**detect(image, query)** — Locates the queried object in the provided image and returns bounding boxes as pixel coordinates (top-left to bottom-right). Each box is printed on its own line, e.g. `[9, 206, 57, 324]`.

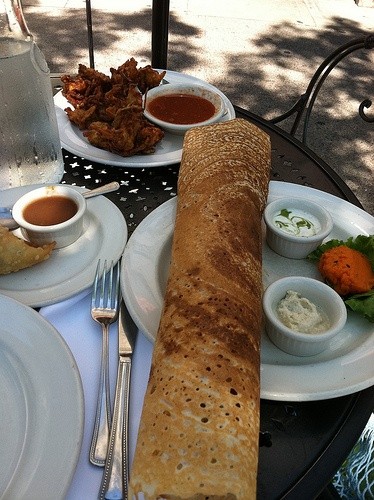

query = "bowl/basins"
[13, 186, 86, 248]
[142, 83, 224, 134]
[263, 277, 348, 356]
[263, 198, 334, 259]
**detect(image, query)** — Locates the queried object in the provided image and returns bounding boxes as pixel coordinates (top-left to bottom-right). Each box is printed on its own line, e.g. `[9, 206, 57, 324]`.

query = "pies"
[124, 118, 272, 500]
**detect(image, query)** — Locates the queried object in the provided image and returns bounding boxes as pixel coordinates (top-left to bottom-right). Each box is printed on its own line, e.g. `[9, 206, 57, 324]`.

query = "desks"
[48, 73, 374, 500]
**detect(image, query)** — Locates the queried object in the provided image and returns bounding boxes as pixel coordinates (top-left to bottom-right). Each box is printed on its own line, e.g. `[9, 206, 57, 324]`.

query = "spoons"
[0, 182, 120, 228]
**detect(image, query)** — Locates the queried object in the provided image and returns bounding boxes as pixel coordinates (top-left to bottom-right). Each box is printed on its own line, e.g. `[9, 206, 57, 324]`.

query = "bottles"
[0, 0, 64, 228]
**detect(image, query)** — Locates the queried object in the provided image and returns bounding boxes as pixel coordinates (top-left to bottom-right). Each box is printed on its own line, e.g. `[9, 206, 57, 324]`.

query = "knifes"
[97, 282, 138, 500]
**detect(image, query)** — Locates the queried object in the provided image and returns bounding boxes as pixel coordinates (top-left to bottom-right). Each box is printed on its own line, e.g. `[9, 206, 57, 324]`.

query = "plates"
[0, 294, 85, 500]
[0, 184, 127, 308]
[119, 180, 374, 400]
[52, 69, 236, 167]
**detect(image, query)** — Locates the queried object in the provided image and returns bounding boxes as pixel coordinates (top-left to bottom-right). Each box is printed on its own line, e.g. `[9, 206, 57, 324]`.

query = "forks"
[91, 259, 119, 466]
